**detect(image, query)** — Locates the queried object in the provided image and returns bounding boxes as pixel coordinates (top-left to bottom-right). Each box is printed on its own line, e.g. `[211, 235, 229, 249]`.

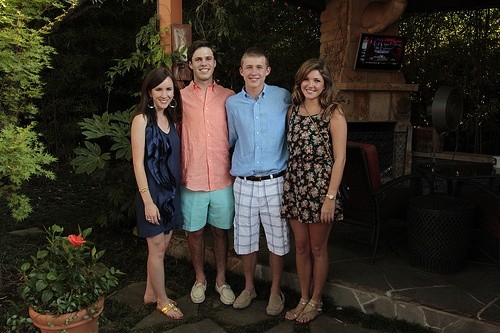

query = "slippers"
[143, 298, 177, 306]
[156, 303, 184, 320]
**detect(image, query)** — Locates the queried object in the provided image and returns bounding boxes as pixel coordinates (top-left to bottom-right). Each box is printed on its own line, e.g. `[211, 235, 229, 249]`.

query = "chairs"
[457, 180, 500, 267]
[333, 145, 415, 265]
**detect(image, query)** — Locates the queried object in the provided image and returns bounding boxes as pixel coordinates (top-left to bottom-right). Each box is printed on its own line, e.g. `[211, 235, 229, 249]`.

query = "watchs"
[138, 186, 151, 195]
[326, 192, 337, 201]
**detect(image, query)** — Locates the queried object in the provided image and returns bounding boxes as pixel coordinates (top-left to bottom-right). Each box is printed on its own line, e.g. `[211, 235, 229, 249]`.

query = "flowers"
[20, 223, 125, 324]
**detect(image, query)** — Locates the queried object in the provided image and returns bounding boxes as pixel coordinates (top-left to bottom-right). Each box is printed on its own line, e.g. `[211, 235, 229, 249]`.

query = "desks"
[427, 160, 495, 200]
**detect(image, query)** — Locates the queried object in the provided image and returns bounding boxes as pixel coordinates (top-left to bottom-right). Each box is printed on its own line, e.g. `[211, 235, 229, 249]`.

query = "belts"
[237, 169, 285, 181]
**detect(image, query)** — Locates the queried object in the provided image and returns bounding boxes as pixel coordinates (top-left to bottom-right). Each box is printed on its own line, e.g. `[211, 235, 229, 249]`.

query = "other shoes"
[215, 283, 235, 305]
[266, 292, 285, 316]
[233, 288, 256, 307]
[190, 280, 207, 303]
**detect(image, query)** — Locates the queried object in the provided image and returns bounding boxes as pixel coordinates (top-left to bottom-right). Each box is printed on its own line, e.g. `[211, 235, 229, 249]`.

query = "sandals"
[285, 298, 309, 320]
[296, 299, 323, 323]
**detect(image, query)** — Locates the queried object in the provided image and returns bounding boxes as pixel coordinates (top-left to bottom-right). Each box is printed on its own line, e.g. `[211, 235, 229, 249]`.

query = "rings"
[147, 219, 151, 221]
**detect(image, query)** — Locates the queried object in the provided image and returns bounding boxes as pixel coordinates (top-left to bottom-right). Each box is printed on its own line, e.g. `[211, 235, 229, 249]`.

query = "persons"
[179, 37, 237, 305]
[284, 57, 348, 324]
[130, 68, 184, 320]
[225, 49, 291, 315]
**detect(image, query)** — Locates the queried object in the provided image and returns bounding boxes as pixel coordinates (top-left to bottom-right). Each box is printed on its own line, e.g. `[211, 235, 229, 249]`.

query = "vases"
[29, 296, 104, 333]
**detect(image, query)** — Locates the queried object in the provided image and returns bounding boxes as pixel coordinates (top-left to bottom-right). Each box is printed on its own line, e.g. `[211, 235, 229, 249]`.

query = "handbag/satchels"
[340, 183, 351, 201]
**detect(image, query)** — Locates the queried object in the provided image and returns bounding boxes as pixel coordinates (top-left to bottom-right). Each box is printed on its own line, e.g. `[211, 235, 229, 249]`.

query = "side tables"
[407, 195, 478, 274]
